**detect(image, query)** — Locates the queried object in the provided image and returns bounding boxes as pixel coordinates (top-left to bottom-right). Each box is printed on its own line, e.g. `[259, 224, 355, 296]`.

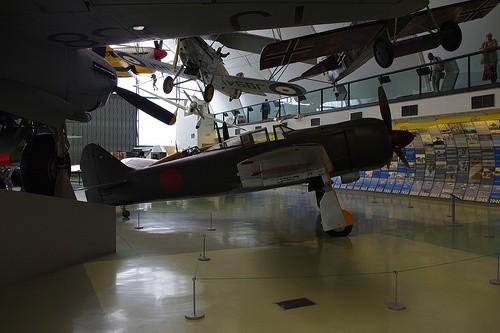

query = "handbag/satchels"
[440, 72, 445, 79]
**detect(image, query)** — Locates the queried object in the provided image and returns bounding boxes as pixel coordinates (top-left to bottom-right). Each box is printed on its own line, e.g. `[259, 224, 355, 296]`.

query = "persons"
[428, 53, 444, 93]
[260, 99, 271, 120]
[479, 33, 499, 84]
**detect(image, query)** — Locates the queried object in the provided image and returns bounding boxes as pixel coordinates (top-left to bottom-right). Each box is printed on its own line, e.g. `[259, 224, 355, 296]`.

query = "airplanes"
[86, 0, 500, 130]
[0, 0, 432, 285]
[80, 85, 418, 237]
